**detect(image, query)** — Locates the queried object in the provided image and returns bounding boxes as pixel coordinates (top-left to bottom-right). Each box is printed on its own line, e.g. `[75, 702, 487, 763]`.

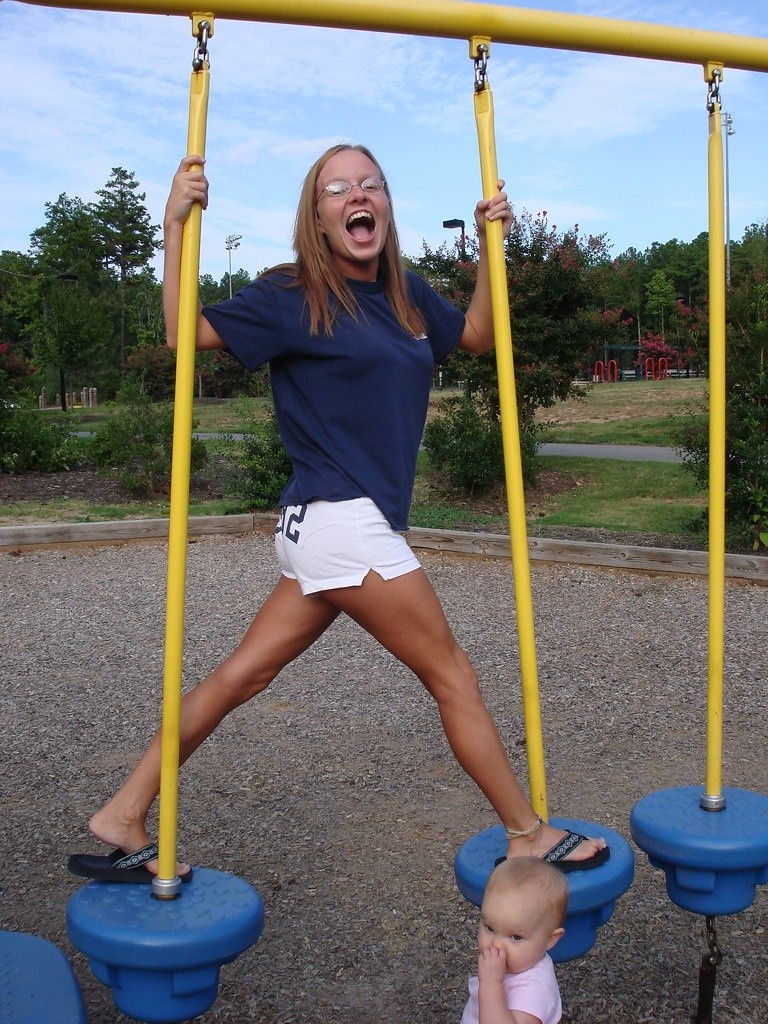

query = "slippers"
[493, 829, 611, 872]
[67, 843, 193, 883]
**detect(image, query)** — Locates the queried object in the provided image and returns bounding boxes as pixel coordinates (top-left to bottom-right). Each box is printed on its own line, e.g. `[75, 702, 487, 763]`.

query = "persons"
[457, 856, 570, 1024]
[67, 144, 611, 884]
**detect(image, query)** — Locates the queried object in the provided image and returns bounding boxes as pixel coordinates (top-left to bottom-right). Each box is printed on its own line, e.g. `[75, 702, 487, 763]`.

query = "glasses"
[314, 177, 394, 199]
